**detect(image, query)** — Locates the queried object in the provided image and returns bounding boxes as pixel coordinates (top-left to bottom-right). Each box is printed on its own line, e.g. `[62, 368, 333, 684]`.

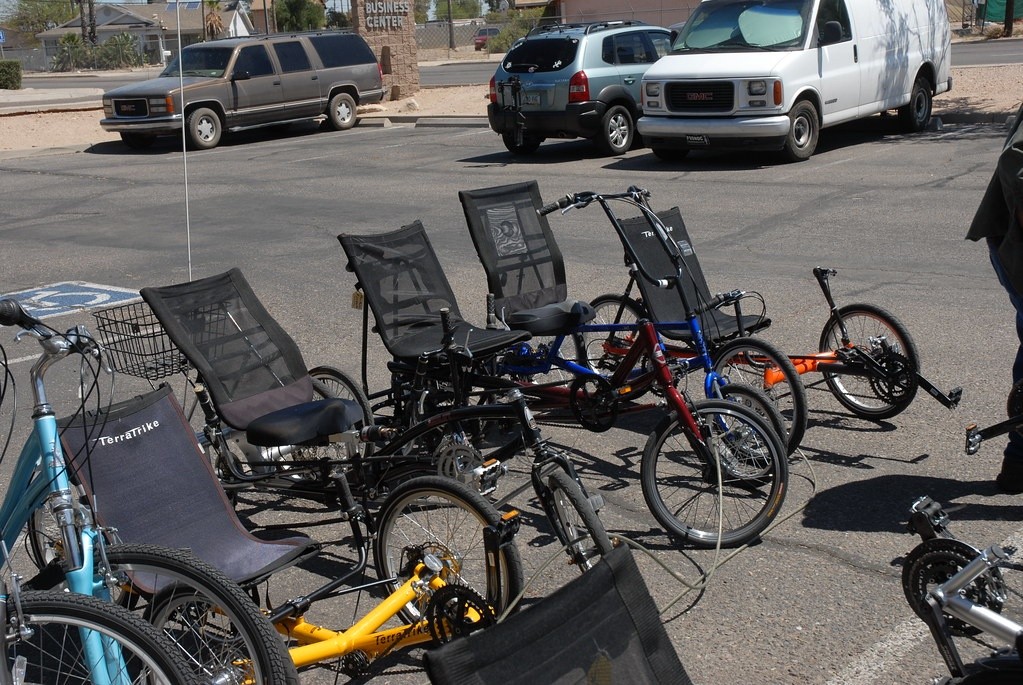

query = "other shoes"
[997, 457, 1023, 492]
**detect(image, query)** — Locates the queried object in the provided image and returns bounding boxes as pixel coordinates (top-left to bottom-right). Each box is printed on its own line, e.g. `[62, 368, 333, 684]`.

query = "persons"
[964, 103, 1023, 495]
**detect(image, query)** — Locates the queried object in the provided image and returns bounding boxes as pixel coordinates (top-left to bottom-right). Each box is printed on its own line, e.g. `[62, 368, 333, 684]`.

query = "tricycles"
[2, 185, 1023, 685]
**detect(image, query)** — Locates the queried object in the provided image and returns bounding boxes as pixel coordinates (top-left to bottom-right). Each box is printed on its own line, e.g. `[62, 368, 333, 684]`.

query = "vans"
[636, 0, 953, 164]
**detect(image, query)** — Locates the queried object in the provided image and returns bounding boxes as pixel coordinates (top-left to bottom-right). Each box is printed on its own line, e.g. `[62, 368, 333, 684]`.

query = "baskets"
[92, 301, 195, 381]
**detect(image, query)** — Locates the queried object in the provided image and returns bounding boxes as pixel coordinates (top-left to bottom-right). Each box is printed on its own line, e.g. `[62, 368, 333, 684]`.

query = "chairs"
[422, 540, 694, 685]
[56, 381, 322, 607]
[616, 206, 773, 347]
[336, 219, 533, 364]
[139, 265, 364, 448]
[616, 46, 635, 64]
[458, 180, 598, 337]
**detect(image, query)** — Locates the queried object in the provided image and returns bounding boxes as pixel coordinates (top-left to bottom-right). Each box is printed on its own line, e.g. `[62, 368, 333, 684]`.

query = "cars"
[650, 19, 704, 55]
[473, 27, 503, 51]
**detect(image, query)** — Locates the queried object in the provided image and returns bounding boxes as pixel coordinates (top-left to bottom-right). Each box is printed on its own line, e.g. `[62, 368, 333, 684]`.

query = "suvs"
[485, 16, 684, 159]
[99, 28, 386, 152]
